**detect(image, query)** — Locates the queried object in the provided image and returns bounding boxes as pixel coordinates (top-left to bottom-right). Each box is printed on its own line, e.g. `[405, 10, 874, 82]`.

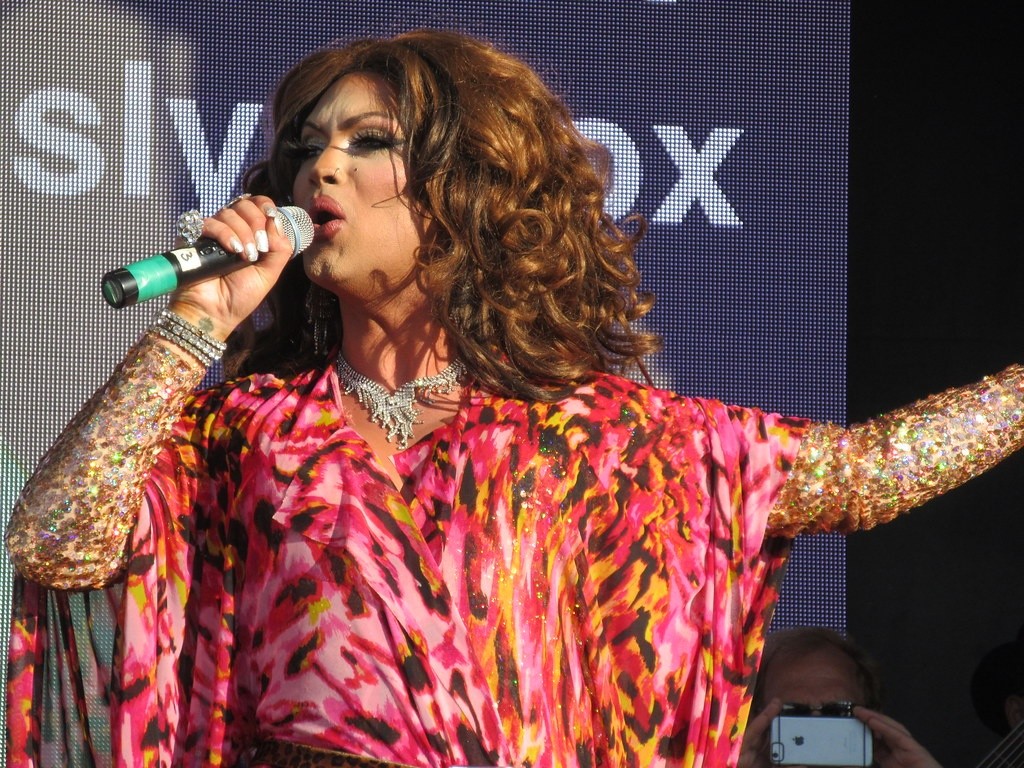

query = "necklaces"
[335, 349, 469, 450]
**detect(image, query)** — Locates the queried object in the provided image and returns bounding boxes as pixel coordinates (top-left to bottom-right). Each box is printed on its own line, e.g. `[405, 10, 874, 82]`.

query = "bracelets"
[149, 306, 229, 367]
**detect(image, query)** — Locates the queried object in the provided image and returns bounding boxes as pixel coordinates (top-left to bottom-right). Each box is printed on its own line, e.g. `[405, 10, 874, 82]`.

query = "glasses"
[779, 700, 855, 718]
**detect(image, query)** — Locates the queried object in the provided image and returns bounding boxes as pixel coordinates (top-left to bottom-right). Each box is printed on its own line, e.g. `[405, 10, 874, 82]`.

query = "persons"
[976, 638, 1024, 768]
[5, 26, 1024, 768]
[735, 627, 945, 768]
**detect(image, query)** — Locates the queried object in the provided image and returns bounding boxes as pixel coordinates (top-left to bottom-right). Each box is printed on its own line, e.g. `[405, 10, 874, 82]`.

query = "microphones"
[101, 206, 314, 308]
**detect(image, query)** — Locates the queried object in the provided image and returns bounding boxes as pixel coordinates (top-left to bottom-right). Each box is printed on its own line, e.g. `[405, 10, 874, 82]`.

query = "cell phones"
[769, 717, 875, 767]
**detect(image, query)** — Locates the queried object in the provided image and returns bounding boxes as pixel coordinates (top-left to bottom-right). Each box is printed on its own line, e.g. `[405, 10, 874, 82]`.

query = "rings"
[219, 191, 251, 208]
[176, 209, 205, 244]
